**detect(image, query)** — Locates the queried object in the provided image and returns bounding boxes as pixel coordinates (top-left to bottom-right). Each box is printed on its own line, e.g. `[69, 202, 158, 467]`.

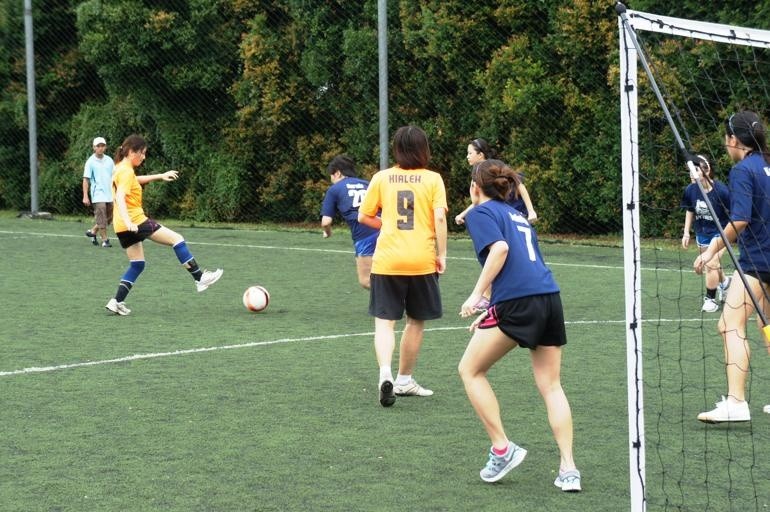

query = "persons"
[357, 125, 449, 407]
[321, 155, 382, 289]
[682, 153, 733, 313]
[458, 158, 581, 492]
[696, 111, 770, 425]
[105, 134, 223, 316]
[454, 137, 537, 310]
[82, 137, 115, 248]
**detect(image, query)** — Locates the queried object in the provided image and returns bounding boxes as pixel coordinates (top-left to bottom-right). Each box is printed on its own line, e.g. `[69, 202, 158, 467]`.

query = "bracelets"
[683, 235, 690, 239]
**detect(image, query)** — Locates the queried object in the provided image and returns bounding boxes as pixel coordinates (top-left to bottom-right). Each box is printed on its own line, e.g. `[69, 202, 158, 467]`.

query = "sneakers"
[105, 298, 132, 315]
[84, 229, 100, 247]
[554, 467, 583, 492]
[378, 374, 396, 407]
[717, 276, 734, 302]
[101, 239, 113, 247]
[194, 268, 224, 292]
[695, 397, 752, 425]
[701, 297, 719, 312]
[393, 377, 434, 397]
[480, 442, 528, 483]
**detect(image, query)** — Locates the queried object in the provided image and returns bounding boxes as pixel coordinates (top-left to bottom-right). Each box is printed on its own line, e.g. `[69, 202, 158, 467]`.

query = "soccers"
[243, 286, 271, 311]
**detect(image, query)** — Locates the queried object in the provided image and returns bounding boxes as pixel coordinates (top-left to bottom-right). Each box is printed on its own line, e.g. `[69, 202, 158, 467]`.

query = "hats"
[92, 136, 107, 146]
